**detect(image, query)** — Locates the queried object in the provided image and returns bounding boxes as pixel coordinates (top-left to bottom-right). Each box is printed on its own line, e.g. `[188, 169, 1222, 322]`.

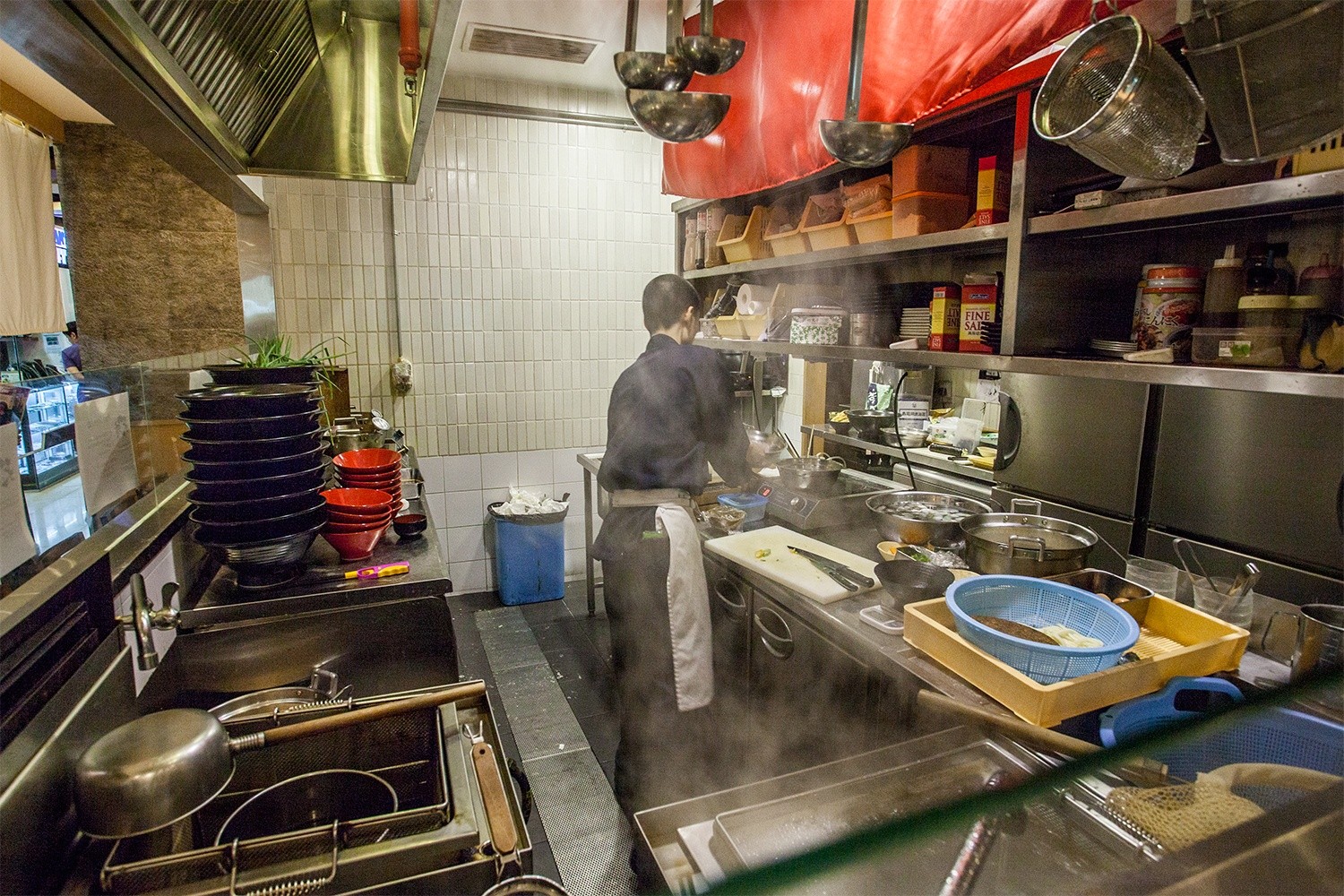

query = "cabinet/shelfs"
[670, 23, 1344, 398]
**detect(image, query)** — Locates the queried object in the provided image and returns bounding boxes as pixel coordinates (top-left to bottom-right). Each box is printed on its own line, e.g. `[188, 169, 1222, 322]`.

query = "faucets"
[935, 766, 1029, 896]
[127, 571, 182, 673]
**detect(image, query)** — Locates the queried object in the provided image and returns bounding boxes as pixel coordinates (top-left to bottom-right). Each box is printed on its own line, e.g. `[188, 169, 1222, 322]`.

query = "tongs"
[1173, 538, 1221, 607]
[1213, 562, 1262, 621]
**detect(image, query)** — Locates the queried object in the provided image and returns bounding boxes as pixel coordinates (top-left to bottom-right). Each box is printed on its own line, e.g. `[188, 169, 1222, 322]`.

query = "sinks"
[96, 701, 478, 896]
[144, 592, 461, 736]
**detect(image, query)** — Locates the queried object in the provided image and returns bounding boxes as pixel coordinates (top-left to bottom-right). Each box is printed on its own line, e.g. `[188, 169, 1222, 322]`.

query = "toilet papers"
[736, 283, 773, 316]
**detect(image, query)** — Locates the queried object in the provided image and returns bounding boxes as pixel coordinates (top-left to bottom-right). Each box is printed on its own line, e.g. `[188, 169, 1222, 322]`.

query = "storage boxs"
[930, 285, 960, 351]
[974, 155, 1012, 226]
[902, 566, 1251, 731]
[958, 282, 1000, 351]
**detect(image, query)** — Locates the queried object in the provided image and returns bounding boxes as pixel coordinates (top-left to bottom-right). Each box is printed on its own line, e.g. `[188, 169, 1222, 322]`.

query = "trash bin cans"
[487, 500, 570, 606]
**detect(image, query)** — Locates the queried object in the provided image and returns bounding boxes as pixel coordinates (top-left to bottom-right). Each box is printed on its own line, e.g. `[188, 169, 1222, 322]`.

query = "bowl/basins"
[1260, 605, 1344, 710]
[891, 145, 970, 239]
[829, 421, 852, 435]
[879, 428, 929, 448]
[1191, 327, 1285, 367]
[174, 362, 427, 588]
[874, 560, 956, 612]
[747, 430, 786, 464]
[844, 410, 897, 435]
[717, 492, 769, 523]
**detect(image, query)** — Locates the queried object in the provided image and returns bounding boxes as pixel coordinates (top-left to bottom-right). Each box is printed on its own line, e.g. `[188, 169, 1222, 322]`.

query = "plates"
[899, 308, 931, 346]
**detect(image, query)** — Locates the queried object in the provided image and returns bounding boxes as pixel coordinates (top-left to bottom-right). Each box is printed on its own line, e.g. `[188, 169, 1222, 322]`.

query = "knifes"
[787, 544, 875, 591]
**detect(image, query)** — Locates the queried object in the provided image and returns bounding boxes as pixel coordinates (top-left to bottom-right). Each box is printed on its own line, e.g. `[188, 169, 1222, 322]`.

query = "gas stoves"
[753, 472, 895, 537]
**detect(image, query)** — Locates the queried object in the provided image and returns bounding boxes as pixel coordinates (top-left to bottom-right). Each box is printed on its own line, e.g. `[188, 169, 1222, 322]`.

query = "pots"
[864, 492, 993, 545]
[73, 669, 486, 876]
[775, 458, 842, 492]
[323, 415, 386, 455]
[959, 498, 1097, 577]
[348, 405, 383, 425]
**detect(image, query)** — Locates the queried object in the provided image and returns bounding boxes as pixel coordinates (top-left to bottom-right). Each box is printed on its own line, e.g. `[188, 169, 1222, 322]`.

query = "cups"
[1124, 558, 1178, 601]
[1192, 576, 1254, 631]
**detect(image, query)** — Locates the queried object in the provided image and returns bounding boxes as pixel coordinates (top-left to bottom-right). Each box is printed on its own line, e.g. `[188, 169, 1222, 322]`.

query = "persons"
[61, 321, 84, 381]
[589, 273, 767, 827]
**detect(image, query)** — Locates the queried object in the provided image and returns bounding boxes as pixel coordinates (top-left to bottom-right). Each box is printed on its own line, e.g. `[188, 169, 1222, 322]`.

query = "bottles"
[1130, 263, 1200, 362]
[695, 206, 706, 269]
[704, 200, 727, 268]
[850, 313, 870, 346]
[683, 209, 697, 271]
[1200, 242, 1341, 367]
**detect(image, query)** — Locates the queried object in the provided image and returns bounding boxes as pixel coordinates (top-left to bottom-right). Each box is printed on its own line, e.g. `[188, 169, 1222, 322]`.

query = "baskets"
[1100, 676, 1344, 818]
[944, 573, 1141, 686]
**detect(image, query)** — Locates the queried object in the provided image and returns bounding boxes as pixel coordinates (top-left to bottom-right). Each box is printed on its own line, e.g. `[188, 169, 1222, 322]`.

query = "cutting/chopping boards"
[705, 525, 884, 604]
